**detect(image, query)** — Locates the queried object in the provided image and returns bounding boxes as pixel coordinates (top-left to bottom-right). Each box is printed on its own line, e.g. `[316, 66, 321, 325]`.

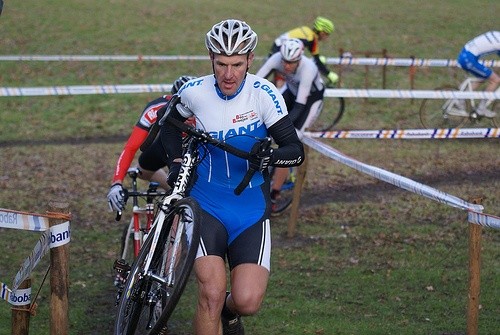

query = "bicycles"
[114, 80, 346, 302]
[112, 95, 274, 334]
[419, 62, 500, 129]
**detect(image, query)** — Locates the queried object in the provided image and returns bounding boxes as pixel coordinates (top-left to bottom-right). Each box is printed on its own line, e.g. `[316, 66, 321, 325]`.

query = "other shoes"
[271, 198, 279, 213]
[154, 306, 168, 335]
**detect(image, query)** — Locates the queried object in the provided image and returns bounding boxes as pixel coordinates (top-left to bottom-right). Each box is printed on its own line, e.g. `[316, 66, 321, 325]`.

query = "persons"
[252, 37, 325, 204]
[267, 16, 339, 84]
[457, 30, 500, 118]
[156, 18, 305, 335]
[106, 76, 200, 214]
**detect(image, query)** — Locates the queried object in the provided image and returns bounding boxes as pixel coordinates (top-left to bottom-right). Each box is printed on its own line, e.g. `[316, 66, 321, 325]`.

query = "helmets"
[205, 20, 258, 54]
[280, 37, 304, 61]
[313, 16, 334, 34]
[171, 75, 198, 95]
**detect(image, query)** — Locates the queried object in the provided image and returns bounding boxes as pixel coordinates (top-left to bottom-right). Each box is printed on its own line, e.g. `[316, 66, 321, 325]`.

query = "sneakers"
[475, 107, 497, 118]
[217, 291, 245, 335]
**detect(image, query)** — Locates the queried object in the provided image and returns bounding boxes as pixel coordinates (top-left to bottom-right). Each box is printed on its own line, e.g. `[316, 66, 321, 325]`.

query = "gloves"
[247, 142, 274, 171]
[328, 70, 339, 84]
[319, 55, 326, 64]
[106, 183, 127, 215]
[167, 161, 199, 192]
